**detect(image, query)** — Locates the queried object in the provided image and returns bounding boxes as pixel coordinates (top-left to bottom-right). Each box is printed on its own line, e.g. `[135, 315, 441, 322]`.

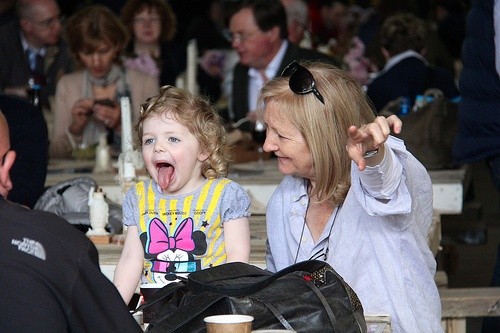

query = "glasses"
[280, 58, 325, 105]
[23, 15, 64, 28]
[222, 29, 270, 43]
[133, 17, 162, 24]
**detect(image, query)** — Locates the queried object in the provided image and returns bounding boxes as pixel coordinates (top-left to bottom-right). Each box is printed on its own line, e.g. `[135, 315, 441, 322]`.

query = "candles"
[186, 40, 197, 94]
[120, 95, 135, 177]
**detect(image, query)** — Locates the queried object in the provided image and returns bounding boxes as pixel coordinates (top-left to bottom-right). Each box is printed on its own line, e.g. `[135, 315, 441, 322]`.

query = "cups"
[204, 315, 254, 333]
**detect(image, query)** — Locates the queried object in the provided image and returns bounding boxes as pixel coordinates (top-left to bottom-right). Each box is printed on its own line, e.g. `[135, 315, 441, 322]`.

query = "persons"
[0, 0, 499, 333]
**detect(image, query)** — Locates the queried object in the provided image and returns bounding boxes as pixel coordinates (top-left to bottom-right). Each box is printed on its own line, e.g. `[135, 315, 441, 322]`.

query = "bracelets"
[363, 146, 380, 159]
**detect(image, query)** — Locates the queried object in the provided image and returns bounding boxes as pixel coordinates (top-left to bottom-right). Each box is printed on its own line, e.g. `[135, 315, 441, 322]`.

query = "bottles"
[398, 94, 433, 116]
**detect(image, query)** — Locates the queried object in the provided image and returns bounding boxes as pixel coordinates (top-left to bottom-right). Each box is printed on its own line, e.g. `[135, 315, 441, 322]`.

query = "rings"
[104, 117, 110, 125]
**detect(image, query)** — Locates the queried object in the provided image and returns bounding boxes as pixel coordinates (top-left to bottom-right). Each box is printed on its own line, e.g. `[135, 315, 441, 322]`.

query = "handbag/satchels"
[141, 260, 367, 333]
[376, 87, 463, 171]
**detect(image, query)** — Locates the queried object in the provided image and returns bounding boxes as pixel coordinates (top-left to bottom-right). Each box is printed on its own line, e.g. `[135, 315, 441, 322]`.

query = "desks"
[35, 161, 464, 216]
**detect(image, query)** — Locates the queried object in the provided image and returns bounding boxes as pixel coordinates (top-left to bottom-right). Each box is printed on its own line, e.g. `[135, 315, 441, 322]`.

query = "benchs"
[439, 288, 500, 333]
